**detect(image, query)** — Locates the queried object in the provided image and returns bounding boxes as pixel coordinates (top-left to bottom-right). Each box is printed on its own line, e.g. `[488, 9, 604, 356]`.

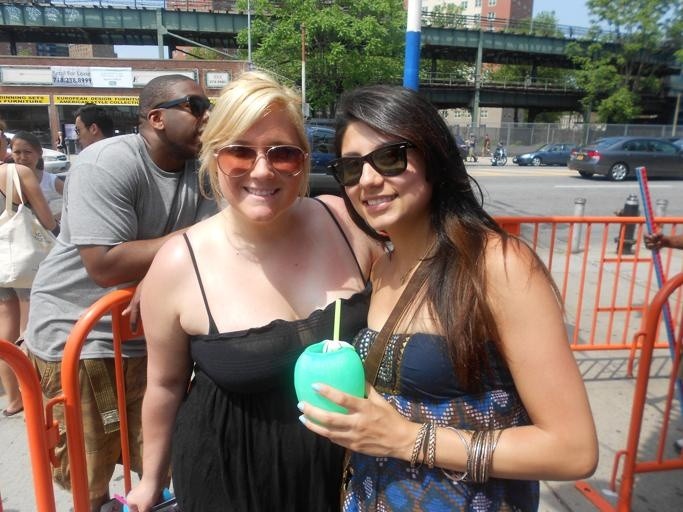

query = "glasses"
[153, 93, 214, 118]
[209, 141, 309, 185]
[73, 126, 84, 134]
[325, 140, 414, 187]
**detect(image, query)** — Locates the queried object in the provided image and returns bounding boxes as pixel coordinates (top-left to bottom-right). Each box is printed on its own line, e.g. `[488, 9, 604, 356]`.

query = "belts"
[76, 354, 129, 437]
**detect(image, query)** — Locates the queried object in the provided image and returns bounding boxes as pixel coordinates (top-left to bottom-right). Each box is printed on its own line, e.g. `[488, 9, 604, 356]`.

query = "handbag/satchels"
[0, 160, 58, 292]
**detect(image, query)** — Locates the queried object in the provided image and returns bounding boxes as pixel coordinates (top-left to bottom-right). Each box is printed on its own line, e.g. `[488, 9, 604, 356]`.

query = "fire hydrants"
[612, 191, 646, 257]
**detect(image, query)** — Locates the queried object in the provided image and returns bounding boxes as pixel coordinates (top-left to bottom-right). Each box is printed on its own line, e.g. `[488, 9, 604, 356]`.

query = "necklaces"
[390, 228, 441, 286]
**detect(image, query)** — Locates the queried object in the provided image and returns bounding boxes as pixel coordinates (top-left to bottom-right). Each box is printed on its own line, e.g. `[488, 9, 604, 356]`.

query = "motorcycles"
[490, 143, 509, 168]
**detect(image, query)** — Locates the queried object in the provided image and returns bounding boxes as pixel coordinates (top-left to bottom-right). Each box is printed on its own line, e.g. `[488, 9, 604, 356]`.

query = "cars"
[303, 124, 344, 198]
[512, 141, 584, 169]
[566, 135, 683, 184]
[657, 135, 683, 150]
[4, 131, 73, 175]
[454, 134, 467, 159]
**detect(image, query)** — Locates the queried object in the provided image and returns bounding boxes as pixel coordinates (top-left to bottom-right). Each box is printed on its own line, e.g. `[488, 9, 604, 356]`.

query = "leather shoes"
[1, 405, 27, 420]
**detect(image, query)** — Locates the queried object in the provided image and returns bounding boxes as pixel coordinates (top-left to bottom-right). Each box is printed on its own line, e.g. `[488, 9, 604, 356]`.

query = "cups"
[293, 342, 367, 427]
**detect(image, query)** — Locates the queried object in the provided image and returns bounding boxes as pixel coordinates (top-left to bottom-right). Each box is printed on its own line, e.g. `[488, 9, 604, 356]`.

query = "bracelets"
[410, 422, 509, 486]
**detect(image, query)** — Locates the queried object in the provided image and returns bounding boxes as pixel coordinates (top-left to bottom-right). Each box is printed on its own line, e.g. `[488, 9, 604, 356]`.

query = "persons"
[481, 133, 493, 157]
[0, 96, 120, 420]
[126, 64, 391, 512]
[24, 74, 222, 512]
[466, 133, 478, 164]
[643, 231, 683, 451]
[300, 84, 599, 512]
